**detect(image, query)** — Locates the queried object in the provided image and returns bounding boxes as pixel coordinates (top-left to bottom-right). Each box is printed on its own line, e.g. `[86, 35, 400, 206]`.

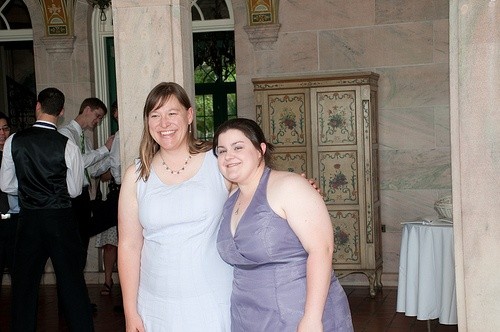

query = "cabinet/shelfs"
[250, 71, 384, 299]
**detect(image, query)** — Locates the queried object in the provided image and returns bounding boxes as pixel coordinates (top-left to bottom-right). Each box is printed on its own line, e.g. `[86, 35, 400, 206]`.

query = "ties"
[81, 130, 91, 186]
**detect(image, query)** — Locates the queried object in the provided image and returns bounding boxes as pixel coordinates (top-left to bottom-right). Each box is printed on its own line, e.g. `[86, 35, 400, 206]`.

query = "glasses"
[0, 126, 10, 131]
[94, 110, 103, 119]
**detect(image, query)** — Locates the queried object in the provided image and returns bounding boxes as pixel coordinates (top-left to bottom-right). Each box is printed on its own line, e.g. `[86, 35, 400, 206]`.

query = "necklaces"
[235, 188, 252, 215]
[159, 151, 191, 174]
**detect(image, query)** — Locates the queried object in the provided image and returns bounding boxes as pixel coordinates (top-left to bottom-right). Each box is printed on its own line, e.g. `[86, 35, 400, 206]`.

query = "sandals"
[100, 278, 113, 297]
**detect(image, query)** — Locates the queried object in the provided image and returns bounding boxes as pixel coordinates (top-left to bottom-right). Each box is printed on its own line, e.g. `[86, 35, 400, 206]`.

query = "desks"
[396, 215, 459, 332]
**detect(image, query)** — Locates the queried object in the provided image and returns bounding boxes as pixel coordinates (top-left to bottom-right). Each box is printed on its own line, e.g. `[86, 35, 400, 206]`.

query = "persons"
[212, 117, 354, 332]
[53, 96, 115, 318]
[118, 83, 324, 332]
[2, 88, 95, 332]
[95, 166, 117, 295]
[0, 114, 18, 304]
[91, 99, 121, 185]
